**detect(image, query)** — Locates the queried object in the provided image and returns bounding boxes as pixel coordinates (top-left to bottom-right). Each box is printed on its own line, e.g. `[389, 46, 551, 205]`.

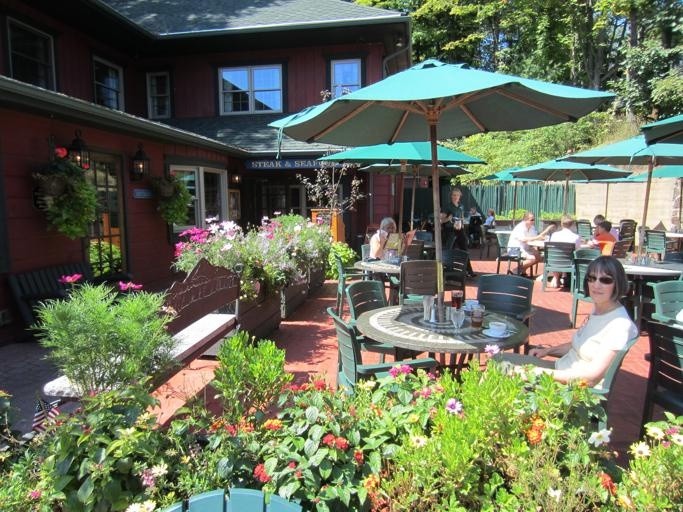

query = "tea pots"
[450, 216, 461, 230]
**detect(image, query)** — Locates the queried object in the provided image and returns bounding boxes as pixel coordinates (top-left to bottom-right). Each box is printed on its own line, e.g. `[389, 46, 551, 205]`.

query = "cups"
[489, 321, 506, 336]
[445, 306, 456, 321]
[421, 295, 434, 323]
[450, 290, 463, 308]
[465, 300, 478, 310]
[471, 304, 485, 328]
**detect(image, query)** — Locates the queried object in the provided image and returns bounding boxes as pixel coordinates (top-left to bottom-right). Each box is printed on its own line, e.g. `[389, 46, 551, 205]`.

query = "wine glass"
[450, 309, 465, 340]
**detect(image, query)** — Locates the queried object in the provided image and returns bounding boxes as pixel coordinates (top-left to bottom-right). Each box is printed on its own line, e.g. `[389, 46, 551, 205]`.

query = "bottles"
[427, 303, 437, 323]
[388, 250, 396, 264]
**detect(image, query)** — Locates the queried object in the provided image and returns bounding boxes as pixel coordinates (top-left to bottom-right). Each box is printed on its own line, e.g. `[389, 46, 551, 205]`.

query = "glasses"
[530, 219, 535, 221]
[587, 274, 616, 284]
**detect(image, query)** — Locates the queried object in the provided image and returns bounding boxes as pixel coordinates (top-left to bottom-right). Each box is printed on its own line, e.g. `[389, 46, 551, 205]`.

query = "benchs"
[5, 260, 128, 342]
[39, 256, 241, 400]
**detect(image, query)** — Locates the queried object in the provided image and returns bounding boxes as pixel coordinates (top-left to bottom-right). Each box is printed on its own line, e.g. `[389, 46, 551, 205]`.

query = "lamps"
[69, 129, 91, 169]
[128, 142, 151, 181]
[226, 167, 243, 189]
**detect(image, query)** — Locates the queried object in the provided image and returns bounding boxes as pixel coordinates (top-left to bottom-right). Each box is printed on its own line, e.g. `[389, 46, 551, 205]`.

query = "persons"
[507, 213, 619, 289]
[478, 254, 639, 418]
[439, 187, 477, 279]
[368, 216, 404, 307]
[405, 205, 496, 260]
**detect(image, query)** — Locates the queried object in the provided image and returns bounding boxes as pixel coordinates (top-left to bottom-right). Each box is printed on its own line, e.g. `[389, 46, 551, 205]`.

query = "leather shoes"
[466, 273, 477, 278]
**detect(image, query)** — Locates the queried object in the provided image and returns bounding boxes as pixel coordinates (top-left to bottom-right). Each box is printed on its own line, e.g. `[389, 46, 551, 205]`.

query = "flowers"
[155, 169, 195, 226]
[166, 204, 337, 295]
[32, 140, 98, 242]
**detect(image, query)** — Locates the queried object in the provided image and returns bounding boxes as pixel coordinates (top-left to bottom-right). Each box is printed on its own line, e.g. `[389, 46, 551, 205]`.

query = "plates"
[461, 306, 472, 312]
[482, 328, 511, 339]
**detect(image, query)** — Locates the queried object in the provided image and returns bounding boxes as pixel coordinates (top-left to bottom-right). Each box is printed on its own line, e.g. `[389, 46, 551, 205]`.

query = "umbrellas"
[315, 138, 490, 256]
[357, 162, 473, 231]
[265, 56, 621, 362]
